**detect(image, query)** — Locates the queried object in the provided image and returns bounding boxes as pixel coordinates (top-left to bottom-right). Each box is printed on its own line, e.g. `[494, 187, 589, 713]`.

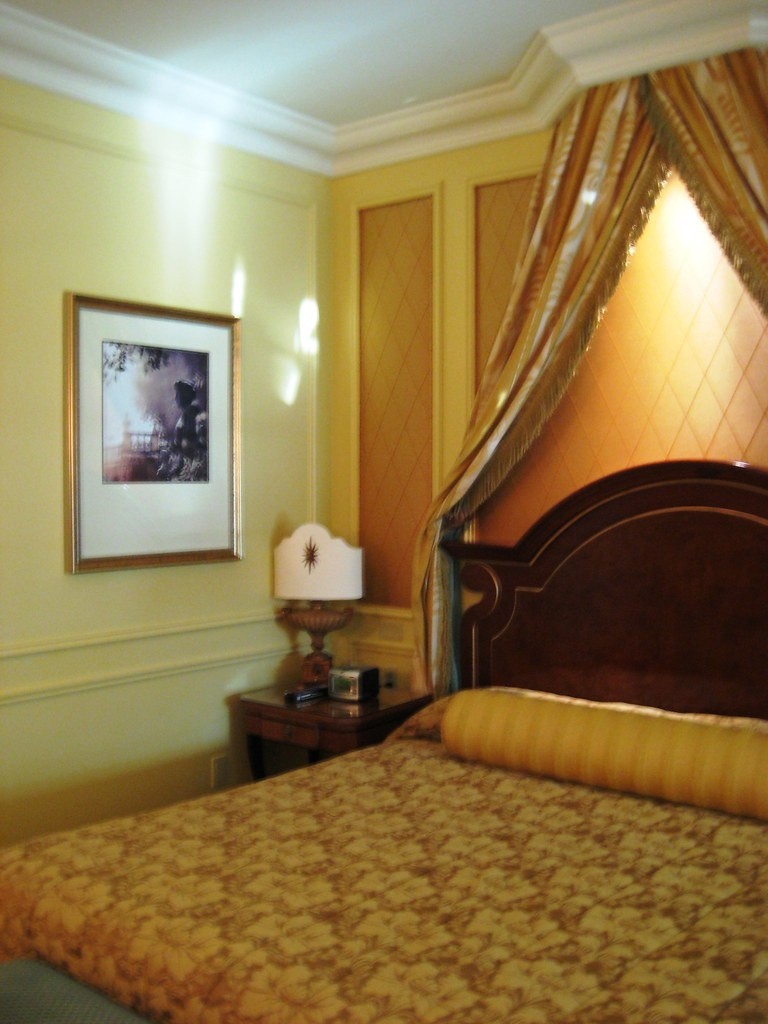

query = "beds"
[0, 456, 767, 1024]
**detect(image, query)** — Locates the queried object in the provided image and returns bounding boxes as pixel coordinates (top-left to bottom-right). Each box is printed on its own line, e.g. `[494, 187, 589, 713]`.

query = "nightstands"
[240, 682, 433, 781]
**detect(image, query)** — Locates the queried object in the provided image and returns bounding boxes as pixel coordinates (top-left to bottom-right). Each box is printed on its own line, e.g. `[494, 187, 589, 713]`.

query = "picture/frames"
[62, 291, 243, 577]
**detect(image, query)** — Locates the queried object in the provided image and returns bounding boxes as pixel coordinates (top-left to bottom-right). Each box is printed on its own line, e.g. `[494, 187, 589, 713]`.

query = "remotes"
[284, 685, 328, 704]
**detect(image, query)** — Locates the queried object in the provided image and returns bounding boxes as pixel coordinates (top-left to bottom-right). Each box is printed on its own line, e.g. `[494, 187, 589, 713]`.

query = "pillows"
[441, 685, 768, 823]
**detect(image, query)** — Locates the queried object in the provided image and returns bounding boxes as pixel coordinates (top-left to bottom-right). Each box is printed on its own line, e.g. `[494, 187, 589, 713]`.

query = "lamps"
[274, 523, 364, 683]
